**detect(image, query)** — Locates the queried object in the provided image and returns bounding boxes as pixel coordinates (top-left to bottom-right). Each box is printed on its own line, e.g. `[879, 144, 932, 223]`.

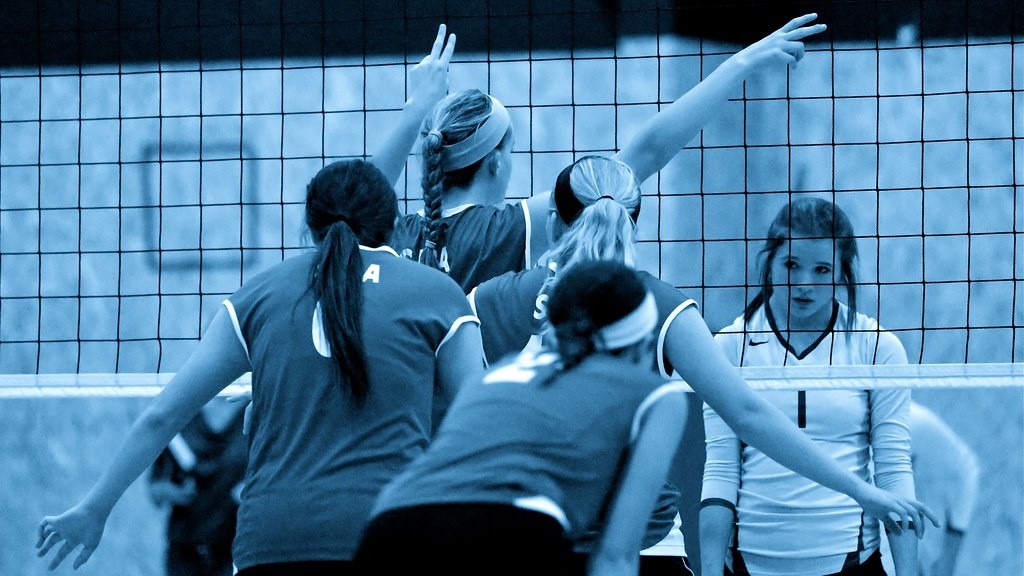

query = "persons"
[35, 159, 483, 576]
[698, 199, 919, 576]
[910, 400, 982, 575]
[350, 260, 687, 576]
[368, 12, 827, 295]
[467, 154, 941, 576]
[147, 398, 250, 576]
